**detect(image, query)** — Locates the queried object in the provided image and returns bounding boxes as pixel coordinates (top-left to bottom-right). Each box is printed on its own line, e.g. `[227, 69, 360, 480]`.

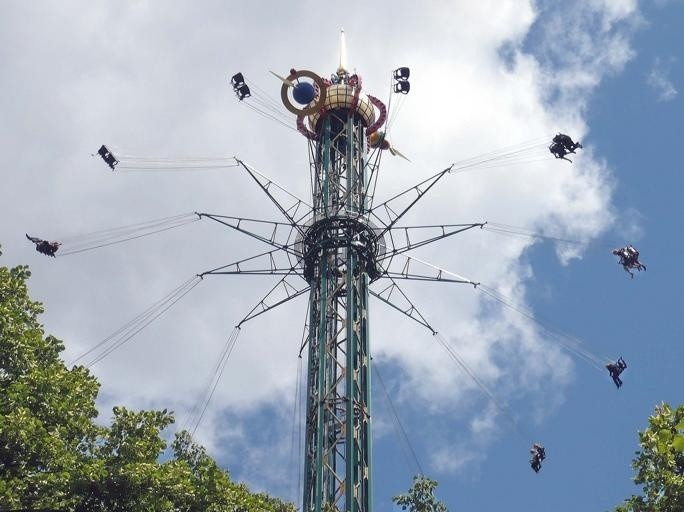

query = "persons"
[613, 244, 646, 278]
[549, 144, 572, 162]
[553, 134, 583, 152]
[605, 363, 623, 389]
[529, 443, 545, 473]
[25, 234, 59, 258]
